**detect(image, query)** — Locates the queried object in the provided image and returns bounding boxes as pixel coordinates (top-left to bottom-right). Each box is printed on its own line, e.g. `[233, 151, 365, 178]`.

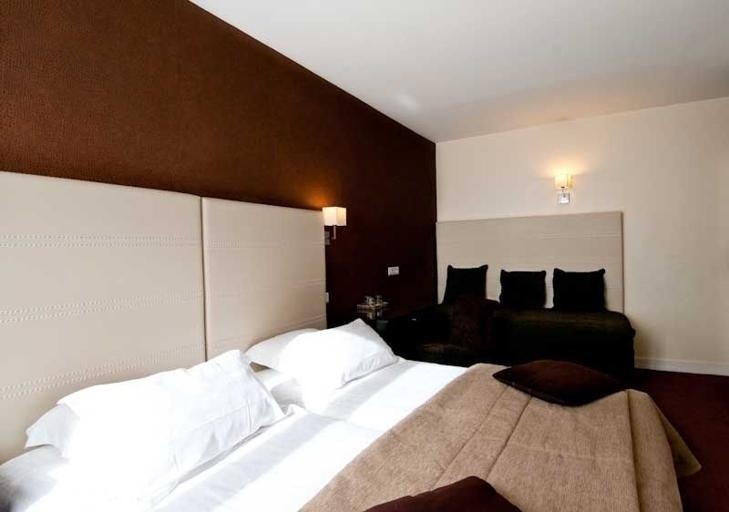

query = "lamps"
[322, 206, 347, 245]
[554, 174, 572, 204]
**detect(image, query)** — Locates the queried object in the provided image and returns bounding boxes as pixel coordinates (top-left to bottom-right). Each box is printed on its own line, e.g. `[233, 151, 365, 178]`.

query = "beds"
[0, 361, 701, 512]
[0, 170, 702, 512]
[383, 212, 636, 385]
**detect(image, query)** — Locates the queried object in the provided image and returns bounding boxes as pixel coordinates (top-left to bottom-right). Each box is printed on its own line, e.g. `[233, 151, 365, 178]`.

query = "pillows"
[442, 265, 488, 303]
[279, 318, 400, 409]
[243, 328, 320, 372]
[24, 368, 186, 483]
[57, 350, 293, 494]
[499, 270, 546, 308]
[552, 269, 609, 311]
